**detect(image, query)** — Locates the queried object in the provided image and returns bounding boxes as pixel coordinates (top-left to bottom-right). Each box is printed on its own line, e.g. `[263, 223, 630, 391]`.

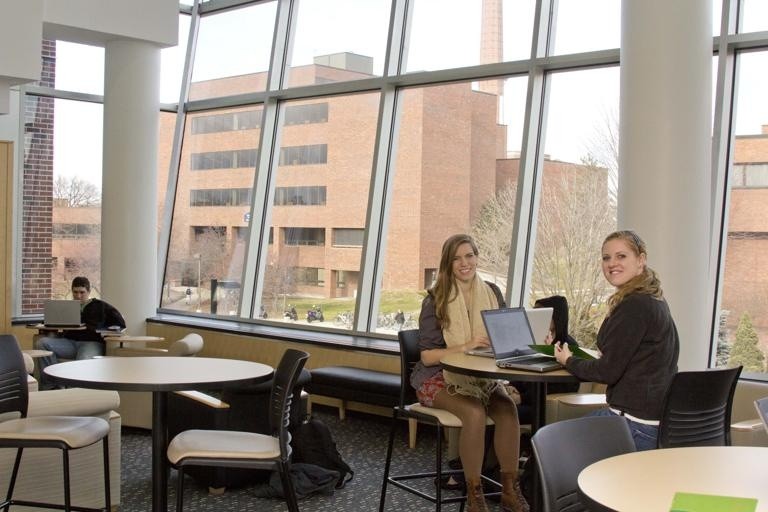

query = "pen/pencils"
[555, 344, 561, 352]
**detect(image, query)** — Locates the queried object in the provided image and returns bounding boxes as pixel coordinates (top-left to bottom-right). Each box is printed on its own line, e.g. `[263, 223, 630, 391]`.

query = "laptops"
[464, 307, 554, 358]
[480, 307, 563, 372]
[44, 299, 86, 327]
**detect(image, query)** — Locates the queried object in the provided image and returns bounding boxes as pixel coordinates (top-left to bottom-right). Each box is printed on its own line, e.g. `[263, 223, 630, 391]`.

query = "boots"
[500, 471, 530, 512]
[466, 481, 489, 512]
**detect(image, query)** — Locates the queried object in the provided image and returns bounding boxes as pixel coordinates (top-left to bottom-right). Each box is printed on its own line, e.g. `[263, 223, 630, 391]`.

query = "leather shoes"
[435, 457, 461, 489]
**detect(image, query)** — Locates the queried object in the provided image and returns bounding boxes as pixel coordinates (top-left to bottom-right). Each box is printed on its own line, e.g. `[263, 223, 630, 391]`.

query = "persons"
[519, 230, 684, 512]
[433, 296, 582, 495]
[394, 308, 405, 331]
[405, 234, 533, 512]
[32, 276, 129, 391]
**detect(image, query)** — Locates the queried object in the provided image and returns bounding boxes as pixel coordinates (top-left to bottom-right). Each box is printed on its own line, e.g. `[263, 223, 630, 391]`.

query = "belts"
[609, 407, 661, 425]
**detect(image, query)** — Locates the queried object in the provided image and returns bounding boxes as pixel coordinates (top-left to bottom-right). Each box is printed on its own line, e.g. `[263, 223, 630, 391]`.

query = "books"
[95, 325, 127, 338]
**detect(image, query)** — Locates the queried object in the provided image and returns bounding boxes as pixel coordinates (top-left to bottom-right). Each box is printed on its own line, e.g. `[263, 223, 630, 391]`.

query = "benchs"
[304, 364, 418, 454]
